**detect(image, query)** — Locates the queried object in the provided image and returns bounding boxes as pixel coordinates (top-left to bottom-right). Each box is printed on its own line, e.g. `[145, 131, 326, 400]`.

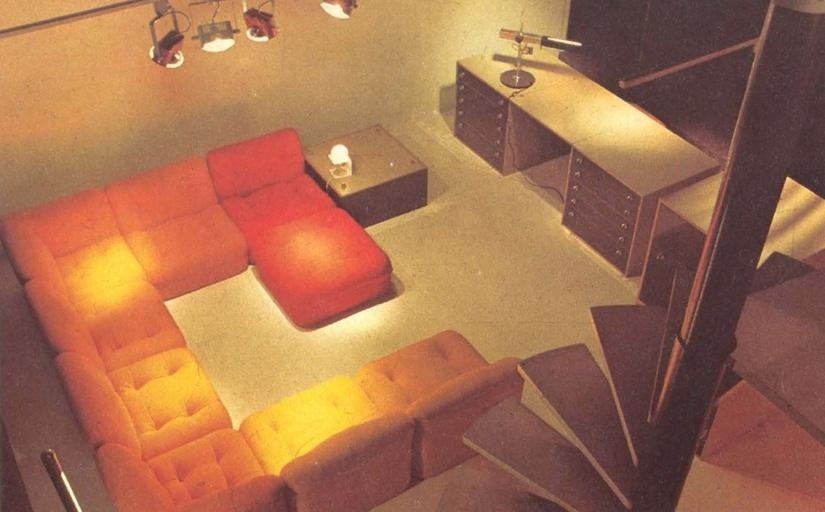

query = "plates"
[305, 126, 429, 229]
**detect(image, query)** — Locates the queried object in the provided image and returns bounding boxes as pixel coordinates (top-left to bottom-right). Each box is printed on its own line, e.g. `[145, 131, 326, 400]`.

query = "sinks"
[0, 129, 524, 512]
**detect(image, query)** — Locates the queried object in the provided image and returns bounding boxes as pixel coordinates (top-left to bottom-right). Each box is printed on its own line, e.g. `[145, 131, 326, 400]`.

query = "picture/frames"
[440, 52, 717, 280]
[635, 174, 825, 313]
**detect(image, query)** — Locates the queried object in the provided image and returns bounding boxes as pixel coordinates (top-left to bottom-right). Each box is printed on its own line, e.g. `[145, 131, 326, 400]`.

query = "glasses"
[320, 2, 355, 24]
[242, 1, 275, 46]
[142, 1, 186, 74]
[327, 144, 353, 181]
[189, 0, 242, 58]
